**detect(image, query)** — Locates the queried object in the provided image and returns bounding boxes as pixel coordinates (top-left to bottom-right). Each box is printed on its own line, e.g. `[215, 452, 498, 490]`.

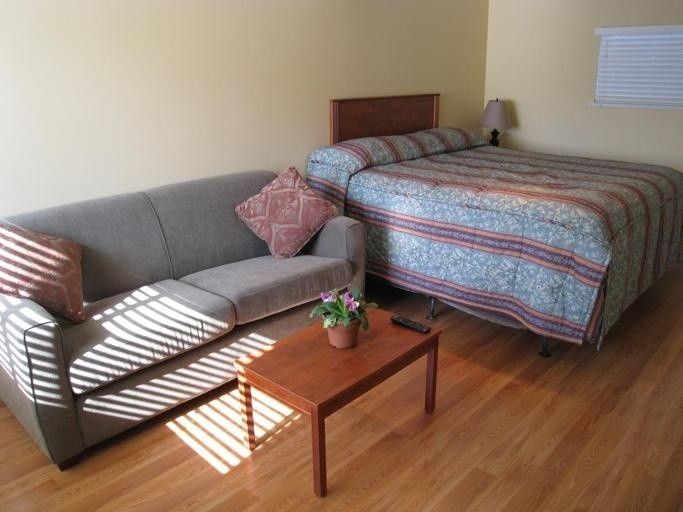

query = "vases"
[328, 319, 361, 349]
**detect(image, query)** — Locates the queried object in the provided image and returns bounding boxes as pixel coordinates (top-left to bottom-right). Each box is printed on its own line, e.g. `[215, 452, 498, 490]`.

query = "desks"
[233, 307, 442, 497]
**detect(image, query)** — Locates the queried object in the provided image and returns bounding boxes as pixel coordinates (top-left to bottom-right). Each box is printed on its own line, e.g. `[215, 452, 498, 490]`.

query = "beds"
[307, 92, 683, 358]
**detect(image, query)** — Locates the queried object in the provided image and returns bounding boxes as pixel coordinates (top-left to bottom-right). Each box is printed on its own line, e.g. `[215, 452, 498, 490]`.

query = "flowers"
[310, 288, 378, 330]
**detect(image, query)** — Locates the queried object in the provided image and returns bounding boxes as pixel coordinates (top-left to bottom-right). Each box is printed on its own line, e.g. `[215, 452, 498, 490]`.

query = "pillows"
[234, 166, 339, 258]
[1, 219, 86, 323]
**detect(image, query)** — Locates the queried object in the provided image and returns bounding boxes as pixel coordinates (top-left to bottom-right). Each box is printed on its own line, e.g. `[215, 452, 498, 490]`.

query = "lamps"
[477, 98, 512, 146]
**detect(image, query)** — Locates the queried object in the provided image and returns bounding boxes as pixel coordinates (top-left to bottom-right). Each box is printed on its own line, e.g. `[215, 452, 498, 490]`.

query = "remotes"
[390, 313, 431, 334]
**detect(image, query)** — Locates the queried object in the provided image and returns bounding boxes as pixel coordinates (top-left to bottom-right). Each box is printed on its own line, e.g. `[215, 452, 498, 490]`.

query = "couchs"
[0, 170, 367, 472]
